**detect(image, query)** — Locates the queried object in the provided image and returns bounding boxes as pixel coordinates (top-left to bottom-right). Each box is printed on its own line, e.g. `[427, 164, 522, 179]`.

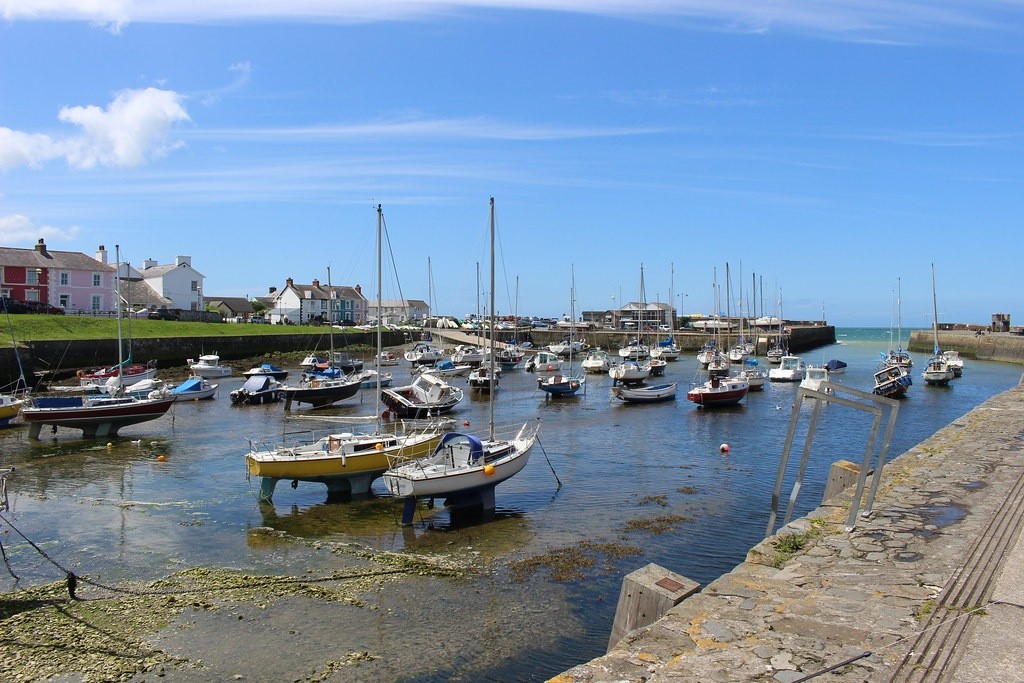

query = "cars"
[603, 323, 638, 332]
[147, 309, 183, 321]
[462, 312, 561, 332]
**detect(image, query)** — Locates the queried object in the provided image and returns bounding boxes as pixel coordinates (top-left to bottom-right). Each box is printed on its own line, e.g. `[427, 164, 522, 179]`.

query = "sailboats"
[0, 284, 32, 431]
[525, 263, 590, 356]
[688, 261, 794, 408]
[608, 263, 653, 382]
[822, 302, 847, 373]
[246, 205, 452, 479]
[581, 307, 618, 374]
[21, 243, 184, 438]
[921, 262, 956, 386]
[619, 262, 682, 378]
[872, 277, 915, 400]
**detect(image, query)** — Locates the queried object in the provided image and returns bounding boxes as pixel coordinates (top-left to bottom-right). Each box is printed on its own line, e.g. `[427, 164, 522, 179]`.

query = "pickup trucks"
[224, 315, 270, 324]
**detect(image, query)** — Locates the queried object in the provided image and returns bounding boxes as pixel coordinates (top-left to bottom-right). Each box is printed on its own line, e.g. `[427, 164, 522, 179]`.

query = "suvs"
[659, 325, 671, 333]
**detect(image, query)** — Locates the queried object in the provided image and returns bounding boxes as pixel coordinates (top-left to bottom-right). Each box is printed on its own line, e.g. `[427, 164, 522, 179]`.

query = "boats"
[374, 195, 537, 499]
[381, 373, 465, 421]
[147, 375, 215, 404]
[242, 364, 289, 381]
[942, 350, 963, 377]
[524, 350, 586, 405]
[612, 381, 677, 403]
[187, 345, 233, 379]
[230, 375, 285, 406]
[769, 353, 808, 382]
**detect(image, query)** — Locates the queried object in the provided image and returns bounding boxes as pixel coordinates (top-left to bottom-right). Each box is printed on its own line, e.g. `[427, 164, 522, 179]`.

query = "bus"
[620, 320, 661, 330]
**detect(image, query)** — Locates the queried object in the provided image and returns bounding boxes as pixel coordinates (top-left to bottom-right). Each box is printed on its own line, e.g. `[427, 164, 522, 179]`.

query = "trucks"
[556, 316, 591, 331]
